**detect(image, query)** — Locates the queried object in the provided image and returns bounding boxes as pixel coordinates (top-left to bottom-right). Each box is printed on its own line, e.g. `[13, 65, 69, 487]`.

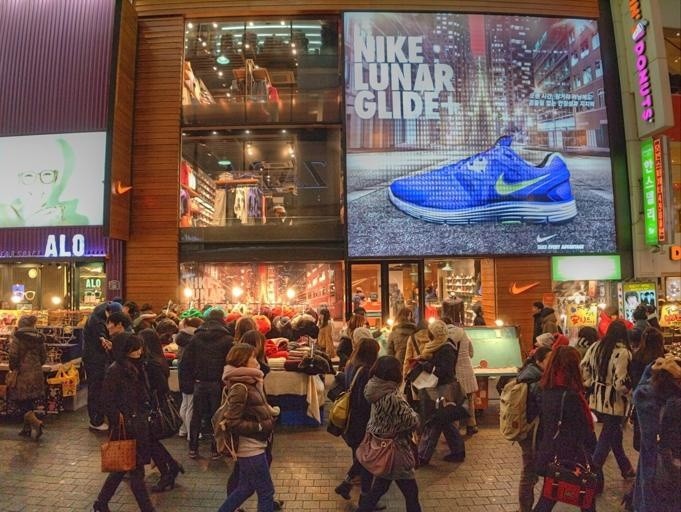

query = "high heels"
[168, 462, 185, 477]
[152, 475, 174, 491]
[93, 501, 110, 512]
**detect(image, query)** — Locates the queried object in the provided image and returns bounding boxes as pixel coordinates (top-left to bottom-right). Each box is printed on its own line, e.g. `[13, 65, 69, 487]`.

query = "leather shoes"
[89, 423, 109, 431]
[443, 452, 465, 461]
[467, 426, 478, 435]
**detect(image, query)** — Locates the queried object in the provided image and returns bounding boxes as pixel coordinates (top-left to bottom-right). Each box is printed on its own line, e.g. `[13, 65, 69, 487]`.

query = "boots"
[335, 481, 352, 499]
[26, 412, 44, 439]
[19, 416, 31, 436]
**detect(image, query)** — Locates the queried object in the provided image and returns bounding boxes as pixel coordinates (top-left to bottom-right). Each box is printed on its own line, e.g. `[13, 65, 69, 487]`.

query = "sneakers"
[388, 135, 578, 226]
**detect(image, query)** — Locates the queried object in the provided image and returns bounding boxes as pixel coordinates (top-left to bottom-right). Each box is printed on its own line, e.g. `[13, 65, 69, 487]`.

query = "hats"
[18, 315, 36, 329]
[428, 320, 448, 339]
[536, 333, 554, 346]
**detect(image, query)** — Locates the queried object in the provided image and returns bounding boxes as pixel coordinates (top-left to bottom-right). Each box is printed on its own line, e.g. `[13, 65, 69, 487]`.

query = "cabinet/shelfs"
[0, 324, 88, 412]
[445, 276, 477, 327]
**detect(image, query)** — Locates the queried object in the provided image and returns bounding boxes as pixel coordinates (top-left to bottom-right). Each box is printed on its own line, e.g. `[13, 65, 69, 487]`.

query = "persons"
[5, 314, 47, 442]
[621, 357, 681, 512]
[327, 337, 386, 510]
[93, 334, 155, 512]
[83, 297, 334, 459]
[211, 343, 281, 512]
[356, 356, 422, 512]
[531, 297, 665, 493]
[227, 330, 284, 512]
[534, 346, 598, 512]
[334, 283, 486, 468]
[500, 347, 555, 512]
[137, 328, 185, 492]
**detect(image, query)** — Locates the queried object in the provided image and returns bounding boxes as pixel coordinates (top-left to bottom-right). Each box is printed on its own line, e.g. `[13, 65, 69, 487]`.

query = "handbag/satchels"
[412, 366, 471, 425]
[5, 368, 19, 389]
[101, 413, 137, 472]
[330, 391, 351, 428]
[543, 459, 598, 509]
[355, 431, 395, 476]
[150, 389, 183, 440]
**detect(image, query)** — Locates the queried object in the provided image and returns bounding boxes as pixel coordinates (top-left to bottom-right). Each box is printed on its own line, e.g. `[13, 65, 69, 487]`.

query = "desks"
[169, 369, 327, 426]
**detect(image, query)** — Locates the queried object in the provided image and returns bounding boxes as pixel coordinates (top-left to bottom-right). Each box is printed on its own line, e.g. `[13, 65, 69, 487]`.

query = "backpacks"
[500, 378, 540, 451]
[211, 383, 248, 457]
